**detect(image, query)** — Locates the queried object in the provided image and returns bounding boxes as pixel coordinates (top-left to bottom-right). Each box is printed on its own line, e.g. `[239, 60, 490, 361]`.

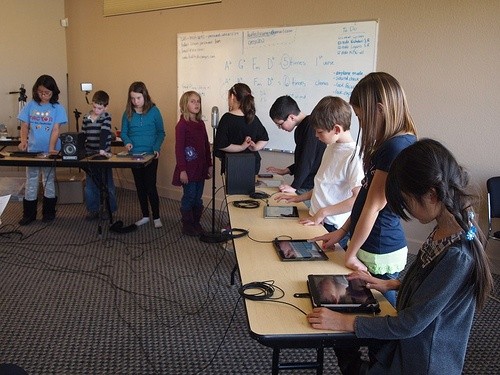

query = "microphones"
[211, 106, 219, 127]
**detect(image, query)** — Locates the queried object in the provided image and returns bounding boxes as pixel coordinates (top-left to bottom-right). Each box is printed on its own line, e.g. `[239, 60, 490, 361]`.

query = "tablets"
[117, 151, 147, 156]
[272, 239, 328, 263]
[11, 151, 42, 155]
[263, 205, 300, 220]
[307, 273, 380, 309]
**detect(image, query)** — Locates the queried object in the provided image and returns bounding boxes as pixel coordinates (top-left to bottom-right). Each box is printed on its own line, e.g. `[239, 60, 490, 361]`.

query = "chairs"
[479, 177, 500, 303]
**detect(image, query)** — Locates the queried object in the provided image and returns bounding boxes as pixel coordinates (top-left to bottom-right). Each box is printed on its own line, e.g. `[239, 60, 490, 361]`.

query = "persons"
[121, 81, 165, 228]
[172, 91, 213, 236]
[307, 72, 418, 375]
[17, 74, 67, 225]
[81, 90, 117, 219]
[274, 96, 366, 251]
[214, 83, 269, 232]
[307, 137, 493, 375]
[266, 95, 328, 207]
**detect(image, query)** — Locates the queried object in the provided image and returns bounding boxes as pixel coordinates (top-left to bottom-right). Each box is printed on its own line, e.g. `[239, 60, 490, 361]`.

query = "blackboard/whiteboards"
[176, 18, 380, 155]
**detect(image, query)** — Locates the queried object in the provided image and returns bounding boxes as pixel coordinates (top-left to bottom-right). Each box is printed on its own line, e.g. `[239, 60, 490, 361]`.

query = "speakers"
[224, 152, 256, 195]
[60, 132, 85, 160]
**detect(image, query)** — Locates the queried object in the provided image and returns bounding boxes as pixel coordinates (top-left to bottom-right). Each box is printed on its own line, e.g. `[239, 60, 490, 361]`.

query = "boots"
[192, 206, 208, 236]
[180, 207, 199, 236]
[41, 195, 57, 222]
[19, 197, 38, 225]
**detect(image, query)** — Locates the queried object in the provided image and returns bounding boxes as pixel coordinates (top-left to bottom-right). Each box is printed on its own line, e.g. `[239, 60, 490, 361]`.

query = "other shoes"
[116, 224, 137, 234]
[100, 211, 110, 219]
[110, 220, 123, 232]
[85, 211, 98, 220]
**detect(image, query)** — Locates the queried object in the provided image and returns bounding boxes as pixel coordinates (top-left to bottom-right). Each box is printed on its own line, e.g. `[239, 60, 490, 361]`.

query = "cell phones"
[259, 173, 273, 178]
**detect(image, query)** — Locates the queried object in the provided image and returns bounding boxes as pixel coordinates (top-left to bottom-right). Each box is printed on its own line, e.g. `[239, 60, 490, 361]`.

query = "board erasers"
[272, 148, 281, 152]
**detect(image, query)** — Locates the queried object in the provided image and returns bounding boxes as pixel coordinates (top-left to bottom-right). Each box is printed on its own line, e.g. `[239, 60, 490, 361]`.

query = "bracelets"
[340, 227, 347, 233]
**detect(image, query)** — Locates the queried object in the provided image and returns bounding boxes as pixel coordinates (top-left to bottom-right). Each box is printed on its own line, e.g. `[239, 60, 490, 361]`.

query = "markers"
[283, 149, 292, 153]
[262, 147, 269, 150]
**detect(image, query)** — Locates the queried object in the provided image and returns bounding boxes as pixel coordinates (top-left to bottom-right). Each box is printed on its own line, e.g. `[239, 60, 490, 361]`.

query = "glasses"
[38, 91, 52, 96]
[276, 116, 288, 128]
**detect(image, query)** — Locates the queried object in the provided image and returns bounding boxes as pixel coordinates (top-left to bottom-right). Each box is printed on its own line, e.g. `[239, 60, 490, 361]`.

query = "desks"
[0, 152, 155, 238]
[222, 174, 397, 375]
